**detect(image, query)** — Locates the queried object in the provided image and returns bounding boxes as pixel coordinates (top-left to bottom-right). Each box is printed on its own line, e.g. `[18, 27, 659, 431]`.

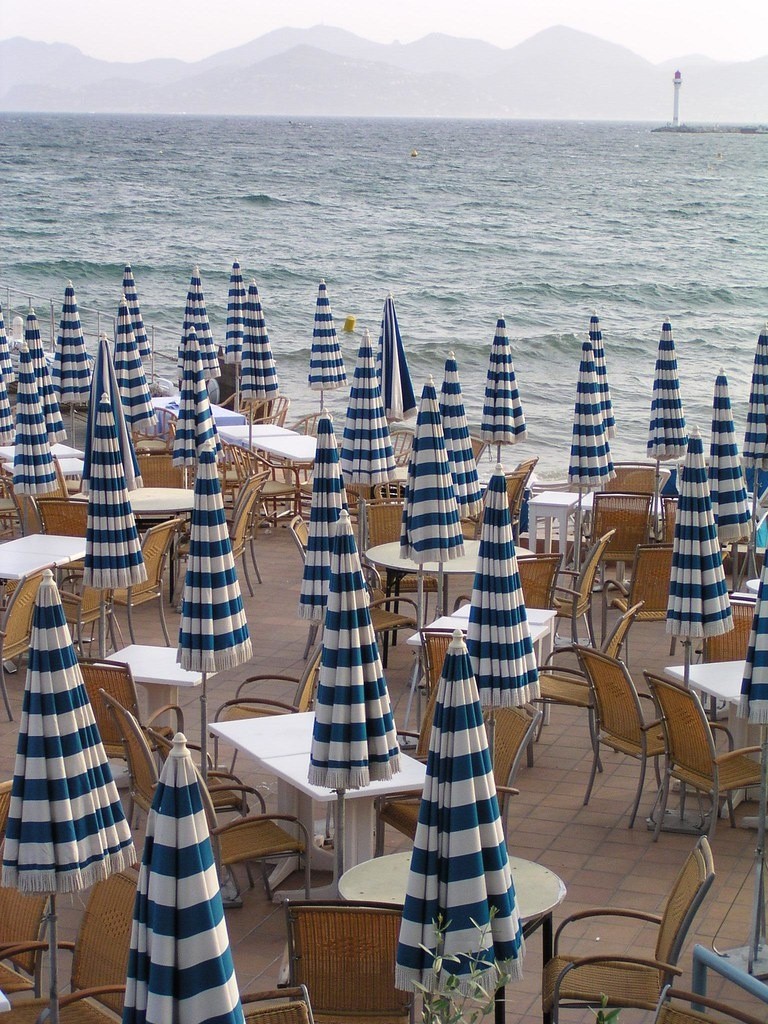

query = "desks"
[216, 423, 299, 517]
[666, 660, 761, 818]
[0, 442, 86, 462]
[150, 396, 247, 463]
[242, 435, 318, 528]
[2, 457, 85, 481]
[0, 534, 95, 644]
[261, 751, 428, 902]
[92, 643, 229, 790]
[338, 850, 566, 1024]
[526, 490, 586, 572]
[451, 603, 558, 725]
[208, 710, 338, 891]
[407, 615, 551, 737]
[744, 578, 760, 594]
[0, 549, 71, 674]
[69, 487, 195, 602]
[365, 539, 537, 669]
[578, 491, 661, 590]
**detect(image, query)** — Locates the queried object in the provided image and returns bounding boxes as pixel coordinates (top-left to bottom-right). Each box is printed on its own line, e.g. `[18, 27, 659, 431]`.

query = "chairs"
[0, 388, 768, 1024]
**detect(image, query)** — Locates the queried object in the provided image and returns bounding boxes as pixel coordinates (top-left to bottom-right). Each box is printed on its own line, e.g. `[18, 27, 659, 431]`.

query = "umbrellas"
[300, 278, 540, 879]
[396, 632, 526, 997]
[122, 731, 245, 1024]
[1, 569, 138, 1024]
[0, 259, 281, 780]
[565, 310, 768, 760]
